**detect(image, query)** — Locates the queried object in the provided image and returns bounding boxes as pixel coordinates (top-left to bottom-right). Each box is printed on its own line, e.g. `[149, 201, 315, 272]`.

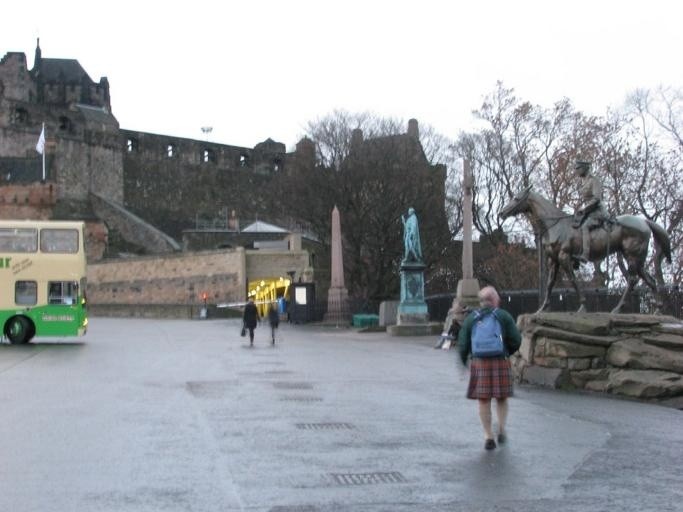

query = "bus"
[0, 219, 89, 344]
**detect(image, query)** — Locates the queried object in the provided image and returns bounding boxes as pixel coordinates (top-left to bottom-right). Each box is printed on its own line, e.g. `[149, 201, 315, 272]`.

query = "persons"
[267, 302, 279, 345]
[433, 318, 461, 348]
[572, 160, 609, 263]
[401, 206, 423, 264]
[242, 298, 261, 346]
[456, 286, 522, 450]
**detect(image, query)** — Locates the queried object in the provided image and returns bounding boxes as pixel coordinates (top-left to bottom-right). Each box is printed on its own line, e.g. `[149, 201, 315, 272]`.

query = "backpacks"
[470, 309, 506, 359]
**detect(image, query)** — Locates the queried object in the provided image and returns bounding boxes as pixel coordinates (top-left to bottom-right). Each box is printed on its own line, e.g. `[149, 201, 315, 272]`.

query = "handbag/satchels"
[240, 322, 248, 338]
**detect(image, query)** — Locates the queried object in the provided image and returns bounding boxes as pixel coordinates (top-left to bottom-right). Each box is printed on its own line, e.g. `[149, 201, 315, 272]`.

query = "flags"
[34, 124, 45, 154]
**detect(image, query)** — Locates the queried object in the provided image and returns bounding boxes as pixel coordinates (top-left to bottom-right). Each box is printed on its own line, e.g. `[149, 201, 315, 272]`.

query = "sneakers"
[497, 433, 507, 444]
[484, 438, 497, 451]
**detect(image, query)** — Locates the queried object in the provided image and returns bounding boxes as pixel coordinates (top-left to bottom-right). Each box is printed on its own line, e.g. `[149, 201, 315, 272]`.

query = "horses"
[496, 183, 673, 315]
